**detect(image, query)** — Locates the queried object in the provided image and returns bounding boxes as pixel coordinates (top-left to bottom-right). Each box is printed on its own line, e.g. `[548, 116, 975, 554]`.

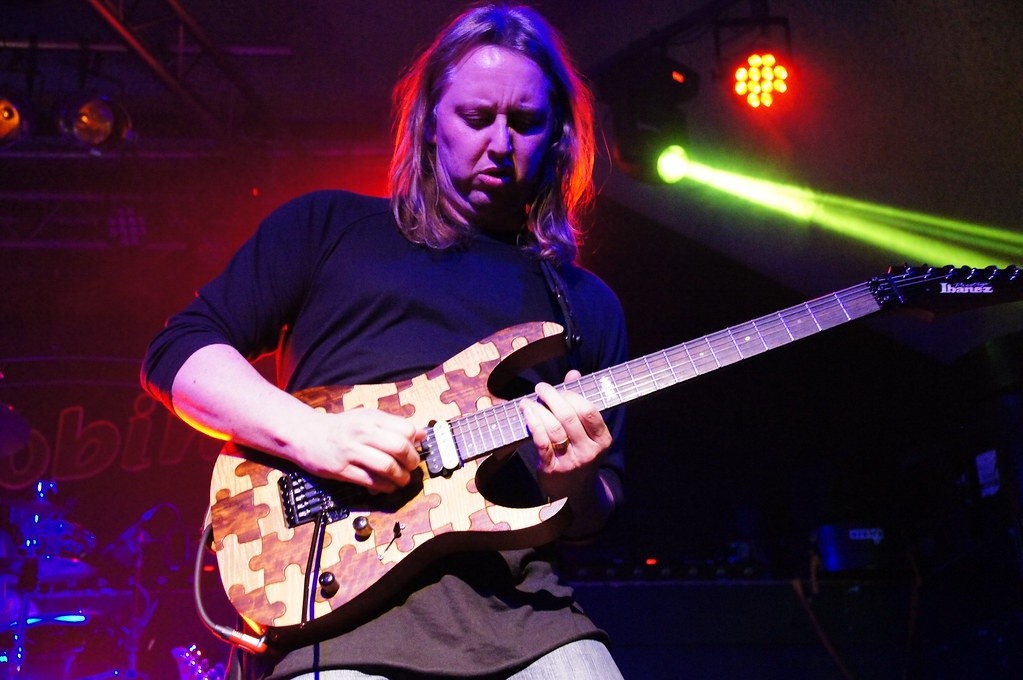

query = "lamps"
[60, 94, 135, 149]
[0, 84, 36, 148]
[708, 16, 802, 125]
[595, 53, 698, 186]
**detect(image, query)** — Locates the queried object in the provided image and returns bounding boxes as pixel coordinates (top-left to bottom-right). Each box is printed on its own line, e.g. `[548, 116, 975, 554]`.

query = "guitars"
[171, 643, 221, 680]
[204, 257, 1023, 650]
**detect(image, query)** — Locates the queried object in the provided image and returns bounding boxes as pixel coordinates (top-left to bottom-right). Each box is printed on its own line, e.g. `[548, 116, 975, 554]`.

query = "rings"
[552, 438, 569, 449]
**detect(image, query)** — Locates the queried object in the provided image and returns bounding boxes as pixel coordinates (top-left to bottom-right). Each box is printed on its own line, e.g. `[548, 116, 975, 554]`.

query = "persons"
[137, 1, 629, 680]
